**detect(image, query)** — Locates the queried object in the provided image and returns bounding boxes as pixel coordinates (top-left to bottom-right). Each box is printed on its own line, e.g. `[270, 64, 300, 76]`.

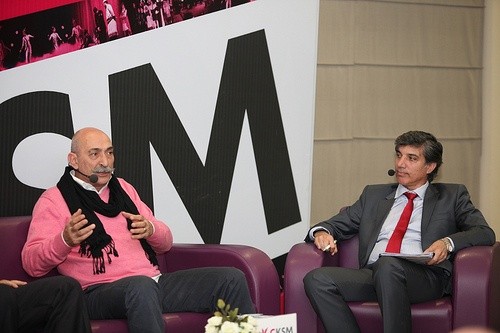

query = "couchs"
[0, 209, 281, 333]
[283, 226, 500, 333]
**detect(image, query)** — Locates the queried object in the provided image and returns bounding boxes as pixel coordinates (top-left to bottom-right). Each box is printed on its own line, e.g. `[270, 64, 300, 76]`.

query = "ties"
[386, 192, 417, 254]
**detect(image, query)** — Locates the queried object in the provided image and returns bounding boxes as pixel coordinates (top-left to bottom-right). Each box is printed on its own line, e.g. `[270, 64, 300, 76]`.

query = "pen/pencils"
[324, 239, 338, 252]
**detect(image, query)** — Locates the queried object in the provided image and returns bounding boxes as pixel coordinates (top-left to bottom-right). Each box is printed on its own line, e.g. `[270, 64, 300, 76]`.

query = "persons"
[0, 0, 256, 72]
[0, 275, 92, 333]
[22, 127, 257, 333]
[304, 131, 496, 333]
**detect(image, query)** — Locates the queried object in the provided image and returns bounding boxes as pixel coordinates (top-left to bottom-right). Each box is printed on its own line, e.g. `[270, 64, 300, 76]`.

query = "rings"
[143, 227, 146, 232]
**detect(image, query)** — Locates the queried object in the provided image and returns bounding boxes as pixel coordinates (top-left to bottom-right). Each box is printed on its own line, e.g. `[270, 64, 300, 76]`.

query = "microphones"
[388, 169, 396, 178]
[69, 165, 99, 183]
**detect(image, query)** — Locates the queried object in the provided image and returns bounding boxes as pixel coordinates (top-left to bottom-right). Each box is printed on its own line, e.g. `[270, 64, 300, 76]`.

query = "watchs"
[440, 238, 453, 255]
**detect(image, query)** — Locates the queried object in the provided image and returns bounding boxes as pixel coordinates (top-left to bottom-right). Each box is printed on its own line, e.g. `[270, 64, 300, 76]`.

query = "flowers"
[204, 298, 262, 333]
